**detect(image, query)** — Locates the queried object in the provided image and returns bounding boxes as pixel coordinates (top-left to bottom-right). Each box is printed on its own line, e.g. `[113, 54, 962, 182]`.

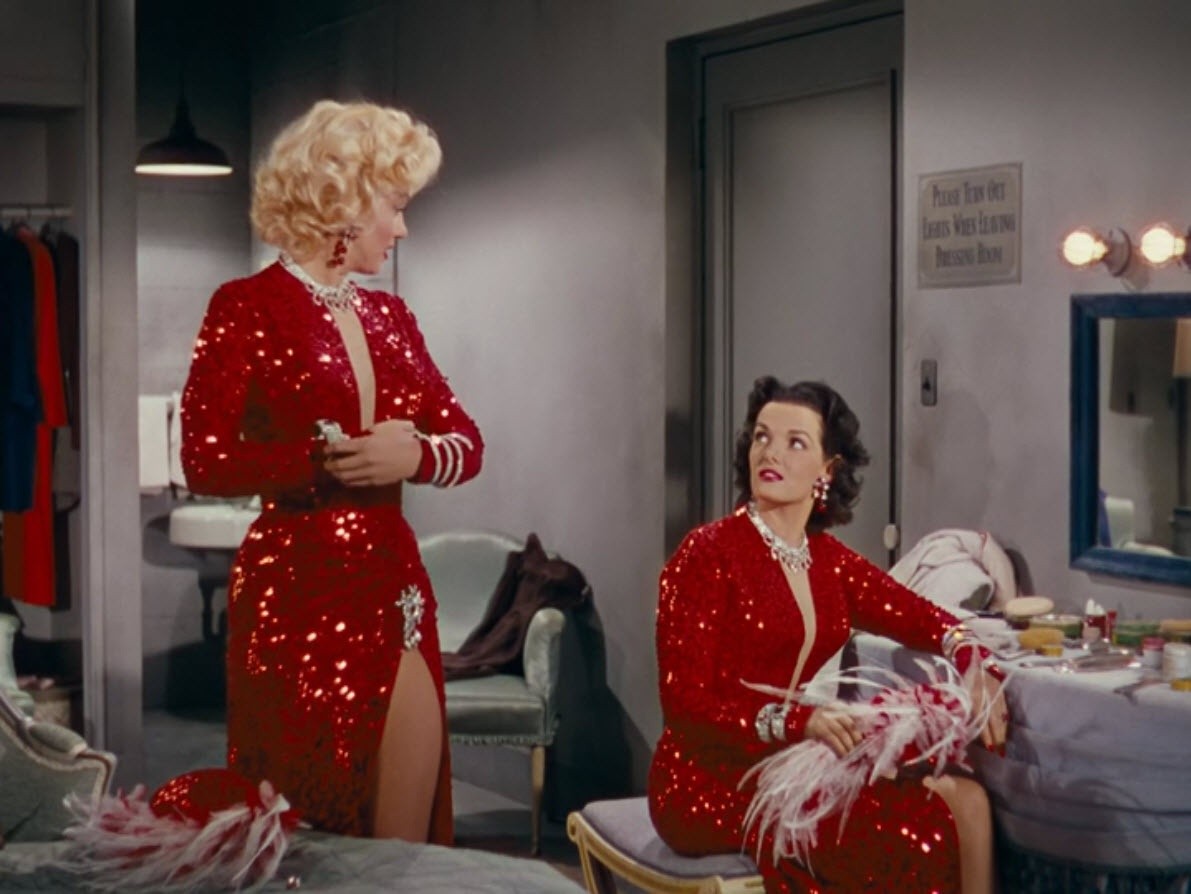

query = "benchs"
[0, 833, 590, 894]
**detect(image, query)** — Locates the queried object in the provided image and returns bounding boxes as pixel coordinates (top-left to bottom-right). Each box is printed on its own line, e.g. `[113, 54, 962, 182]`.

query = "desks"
[835, 608, 1191, 894]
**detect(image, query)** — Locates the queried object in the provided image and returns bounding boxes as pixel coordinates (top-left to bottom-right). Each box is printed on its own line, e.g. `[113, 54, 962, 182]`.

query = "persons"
[646, 375, 1009, 894]
[180, 100, 488, 852]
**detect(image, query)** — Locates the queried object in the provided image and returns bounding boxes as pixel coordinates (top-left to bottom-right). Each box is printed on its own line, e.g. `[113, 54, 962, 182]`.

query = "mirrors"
[1067, 288, 1191, 589]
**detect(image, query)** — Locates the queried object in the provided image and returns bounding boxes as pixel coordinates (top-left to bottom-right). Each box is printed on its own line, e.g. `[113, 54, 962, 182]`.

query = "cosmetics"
[1140, 635, 1191, 684]
[1084, 599, 1119, 647]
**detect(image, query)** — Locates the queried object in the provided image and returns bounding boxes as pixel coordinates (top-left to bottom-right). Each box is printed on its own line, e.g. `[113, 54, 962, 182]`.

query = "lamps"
[134, 83, 233, 179]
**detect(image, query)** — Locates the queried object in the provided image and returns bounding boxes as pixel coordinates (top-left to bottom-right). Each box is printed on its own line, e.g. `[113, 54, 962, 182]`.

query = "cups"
[1083, 613, 1106, 637]
[1162, 642, 1191, 682]
[1142, 637, 1165, 667]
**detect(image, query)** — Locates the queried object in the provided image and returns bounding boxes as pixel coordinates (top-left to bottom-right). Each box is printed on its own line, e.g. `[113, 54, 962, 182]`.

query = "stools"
[566, 794, 766, 894]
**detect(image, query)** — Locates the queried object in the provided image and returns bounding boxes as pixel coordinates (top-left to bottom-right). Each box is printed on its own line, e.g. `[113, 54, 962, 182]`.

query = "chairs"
[416, 530, 567, 857]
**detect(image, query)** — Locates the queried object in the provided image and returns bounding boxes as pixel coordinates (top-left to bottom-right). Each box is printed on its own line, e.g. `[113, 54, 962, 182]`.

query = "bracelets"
[753, 703, 778, 741]
[445, 436, 463, 486]
[438, 440, 454, 484]
[941, 624, 969, 647]
[427, 437, 442, 483]
[943, 631, 981, 657]
[769, 704, 791, 742]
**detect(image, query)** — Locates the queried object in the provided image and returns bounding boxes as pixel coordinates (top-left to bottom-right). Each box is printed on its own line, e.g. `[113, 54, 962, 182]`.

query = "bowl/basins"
[1003, 596, 1082, 638]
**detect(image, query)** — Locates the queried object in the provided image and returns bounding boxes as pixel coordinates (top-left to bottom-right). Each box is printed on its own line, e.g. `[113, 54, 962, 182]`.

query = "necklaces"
[280, 249, 363, 312]
[747, 499, 815, 573]
